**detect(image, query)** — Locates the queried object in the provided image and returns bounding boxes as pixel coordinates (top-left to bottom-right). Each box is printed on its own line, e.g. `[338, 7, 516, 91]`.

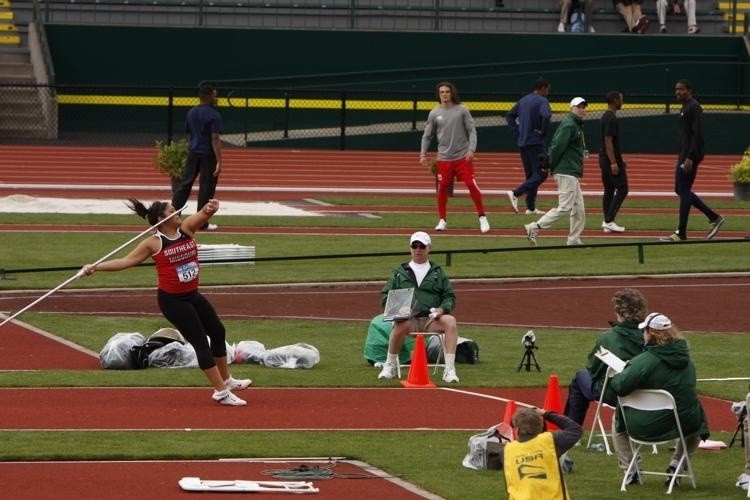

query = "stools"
[395, 330, 445, 378]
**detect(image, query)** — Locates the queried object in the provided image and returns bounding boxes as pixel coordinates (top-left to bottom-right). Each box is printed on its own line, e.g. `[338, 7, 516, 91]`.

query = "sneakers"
[434, 217, 448, 231]
[565, 233, 583, 246]
[211, 388, 248, 407]
[524, 221, 541, 246]
[553, 16, 704, 35]
[507, 189, 521, 214]
[623, 462, 683, 489]
[223, 376, 253, 392]
[525, 208, 546, 216]
[602, 219, 627, 233]
[659, 232, 688, 241]
[377, 361, 397, 381]
[705, 214, 726, 241]
[478, 215, 490, 234]
[441, 366, 459, 383]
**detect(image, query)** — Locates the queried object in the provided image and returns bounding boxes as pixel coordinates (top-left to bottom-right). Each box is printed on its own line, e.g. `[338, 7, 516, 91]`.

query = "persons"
[377, 231, 461, 384]
[659, 79, 725, 244]
[595, 91, 629, 233]
[419, 81, 491, 233]
[173, 82, 224, 233]
[524, 97, 591, 249]
[613, 0, 651, 33]
[78, 198, 251, 408]
[562, 289, 650, 426]
[506, 77, 551, 215]
[557, 1, 596, 33]
[497, 406, 586, 500]
[609, 312, 710, 487]
[655, 0, 703, 35]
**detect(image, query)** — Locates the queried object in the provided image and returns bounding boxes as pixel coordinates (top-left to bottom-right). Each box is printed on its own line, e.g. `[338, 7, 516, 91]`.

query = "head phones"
[646, 313, 664, 333]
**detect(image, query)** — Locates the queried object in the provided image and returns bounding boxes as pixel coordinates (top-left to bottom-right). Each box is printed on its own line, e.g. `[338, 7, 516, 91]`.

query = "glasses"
[410, 243, 426, 250]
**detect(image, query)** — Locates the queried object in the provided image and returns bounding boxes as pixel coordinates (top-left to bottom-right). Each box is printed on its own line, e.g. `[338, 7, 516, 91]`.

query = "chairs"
[587, 362, 618, 456]
[618, 390, 695, 495]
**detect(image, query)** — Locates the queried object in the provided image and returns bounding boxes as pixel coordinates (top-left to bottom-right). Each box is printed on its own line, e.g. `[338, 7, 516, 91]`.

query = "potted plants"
[430, 159, 455, 195]
[153, 137, 192, 194]
[726, 147, 749, 200]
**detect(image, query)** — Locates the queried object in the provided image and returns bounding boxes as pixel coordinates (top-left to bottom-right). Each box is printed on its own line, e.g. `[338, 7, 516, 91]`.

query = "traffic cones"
[542, 375, 565, 434]
[497, 399, 519, 442]
[400, 331, 436, 391]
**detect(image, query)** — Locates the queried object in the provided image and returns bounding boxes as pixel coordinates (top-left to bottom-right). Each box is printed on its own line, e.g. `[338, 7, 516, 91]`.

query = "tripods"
[517, 343, 542, 373]
[729, 417, 745, 447]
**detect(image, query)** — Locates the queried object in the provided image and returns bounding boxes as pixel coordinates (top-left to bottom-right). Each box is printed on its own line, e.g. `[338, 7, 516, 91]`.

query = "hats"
[409, 231, 432, 246]
[637, 312, 674, 332]
[570, 96, 589, 108]
